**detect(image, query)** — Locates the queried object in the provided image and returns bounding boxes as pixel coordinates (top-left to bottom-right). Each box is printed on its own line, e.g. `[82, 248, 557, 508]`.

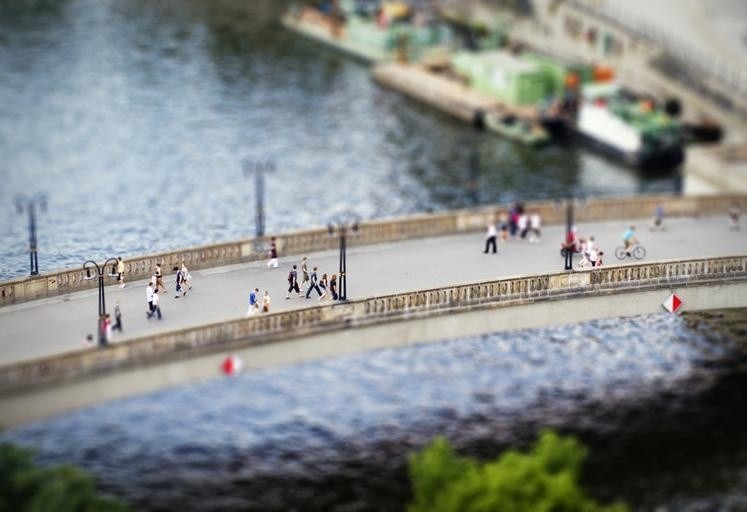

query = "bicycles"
[615, 242, 646, 260]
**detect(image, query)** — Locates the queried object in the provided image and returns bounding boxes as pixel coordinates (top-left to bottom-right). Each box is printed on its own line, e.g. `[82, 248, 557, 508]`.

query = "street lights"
[553, 190, 587, 270]
[82, 257, 120, 345]
[327, 210, 362, 302]
[12, 191, 48, 276]
[241, 153, 276, 236]
